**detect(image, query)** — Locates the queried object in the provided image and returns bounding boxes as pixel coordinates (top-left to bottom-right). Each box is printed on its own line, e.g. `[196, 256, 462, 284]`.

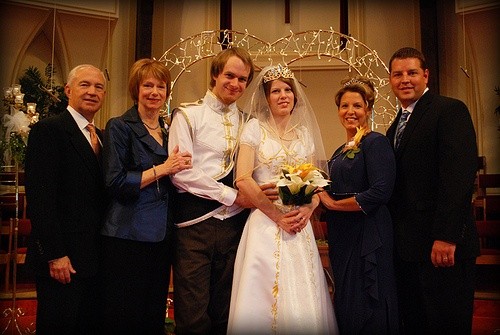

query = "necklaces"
[266, 120, 296, 141]
[141, 121, 160, 130]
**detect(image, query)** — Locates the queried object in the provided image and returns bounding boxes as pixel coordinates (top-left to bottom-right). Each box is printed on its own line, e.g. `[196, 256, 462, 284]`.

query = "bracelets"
[151, 164, 161, 193]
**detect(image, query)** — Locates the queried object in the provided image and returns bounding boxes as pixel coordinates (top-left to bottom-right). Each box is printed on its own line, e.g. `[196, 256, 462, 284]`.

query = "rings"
[302, 216, 307, 222]
[442, 257, 448, 262]
[186, 159, 190, 164]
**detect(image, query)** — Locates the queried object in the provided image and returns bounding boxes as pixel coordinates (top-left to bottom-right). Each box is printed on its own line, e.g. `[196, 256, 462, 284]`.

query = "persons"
[169, 47, 337, 335]
[26, 63, 107, 335]
[302, 78, 404, 335]
[385, 48, 481, 335]
[101, 58, 193, 335]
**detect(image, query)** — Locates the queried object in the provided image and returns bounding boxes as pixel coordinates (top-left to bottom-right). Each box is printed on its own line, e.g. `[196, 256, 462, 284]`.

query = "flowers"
[271, 159, 332, 241]
[339, 125, 367, 160]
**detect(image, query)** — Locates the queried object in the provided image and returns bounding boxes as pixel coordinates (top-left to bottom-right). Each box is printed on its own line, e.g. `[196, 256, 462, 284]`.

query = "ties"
[395, 109, 409, 151]
[85, 123, 101, 162]
